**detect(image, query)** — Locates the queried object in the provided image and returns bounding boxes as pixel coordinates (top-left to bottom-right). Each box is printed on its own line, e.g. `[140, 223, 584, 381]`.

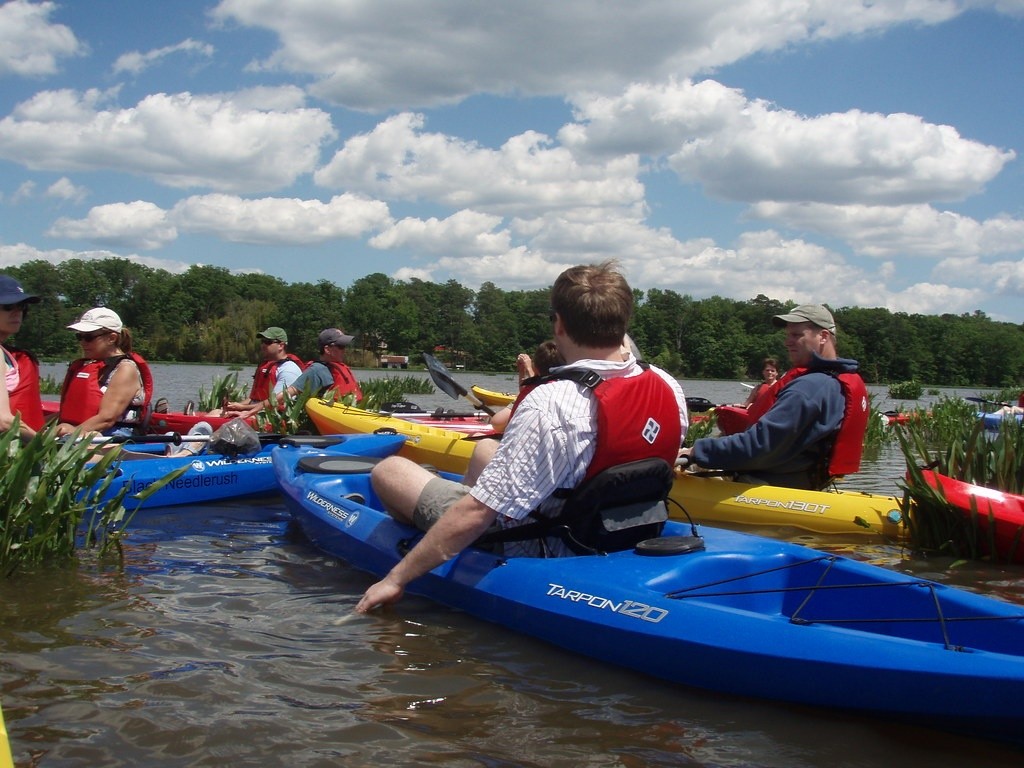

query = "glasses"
[2, 302, 27, 312]
[338, 344, 346, 350]
[75, 332, 108, 342]
[261, 338, 281, 344]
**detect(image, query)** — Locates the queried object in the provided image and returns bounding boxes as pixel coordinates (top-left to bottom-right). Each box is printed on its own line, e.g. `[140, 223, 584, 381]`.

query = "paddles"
[381, 402, 492, 417]
[422, 352, 496, 417]
[740, 382, 755, 389]
[55, 432, 283, 444]
[685, 397, 747, 412]
[674, 454, 695, 469]
[220, 387, 228, 418]
[965, 396, 1013, 407]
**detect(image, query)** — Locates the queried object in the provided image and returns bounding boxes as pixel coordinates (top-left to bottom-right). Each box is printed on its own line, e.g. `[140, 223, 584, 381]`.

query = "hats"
[319, 328, 355, 352]
[0, 275, 41, 306]
[65, 306, 123, 334]
[256, 326, 288, 345]
[773, 304, 835, 336]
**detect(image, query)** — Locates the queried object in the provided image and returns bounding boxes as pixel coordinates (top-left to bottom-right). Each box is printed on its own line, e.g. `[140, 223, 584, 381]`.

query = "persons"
[354, 260, 689, 614]
[744, 358, 778, 408]
[678, 305, 868, 490]
[0, 275, 212, 465]
[155, 327, 363, 424]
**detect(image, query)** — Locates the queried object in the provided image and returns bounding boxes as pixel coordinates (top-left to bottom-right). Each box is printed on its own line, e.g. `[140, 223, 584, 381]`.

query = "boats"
[270, 442, 1023, 733]
[39, 401, 407, 517]
[904, 463, 1024, 573]
[303, 393, 916, 545]
[971, 408, 1024, 434]
[871, 407, 933, 430]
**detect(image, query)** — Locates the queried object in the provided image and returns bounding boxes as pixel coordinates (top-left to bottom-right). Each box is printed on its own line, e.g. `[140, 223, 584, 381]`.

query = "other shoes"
[184, 400, 196, 416]
[165, 422, 213, 455]
[156, 398, 170, 416]
